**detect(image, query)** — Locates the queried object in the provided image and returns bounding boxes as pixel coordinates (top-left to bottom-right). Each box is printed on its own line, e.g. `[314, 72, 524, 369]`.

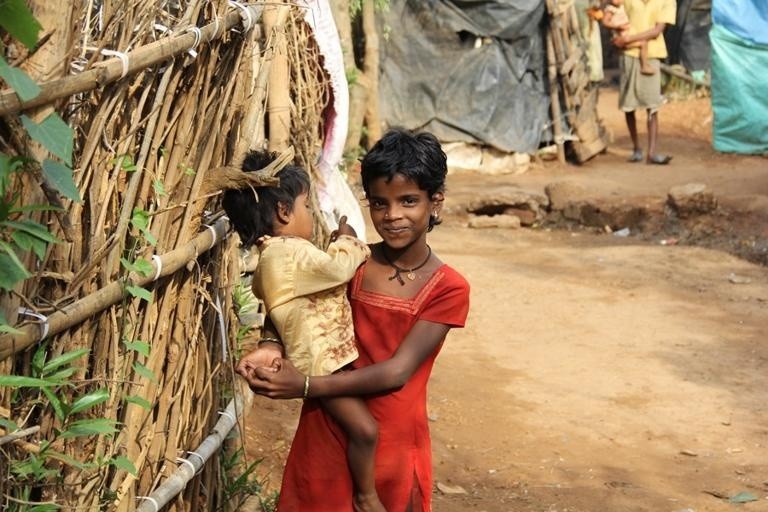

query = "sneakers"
[627, 146, 675, 165]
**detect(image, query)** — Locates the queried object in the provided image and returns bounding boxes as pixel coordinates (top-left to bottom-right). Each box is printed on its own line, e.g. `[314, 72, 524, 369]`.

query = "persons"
[609, 0, 677, 168]
[232, 126, 474, 511]
[222, 147, 390, 512]
[602, 2, 658, 77]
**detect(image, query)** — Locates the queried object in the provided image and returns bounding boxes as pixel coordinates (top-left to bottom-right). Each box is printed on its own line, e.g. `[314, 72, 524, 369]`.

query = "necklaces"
[379, 238, 431, 286]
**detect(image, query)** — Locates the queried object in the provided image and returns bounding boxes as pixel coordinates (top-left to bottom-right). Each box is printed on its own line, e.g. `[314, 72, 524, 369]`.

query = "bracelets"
[302, 373, 311, 402]
[253, 336, 284, 346]
